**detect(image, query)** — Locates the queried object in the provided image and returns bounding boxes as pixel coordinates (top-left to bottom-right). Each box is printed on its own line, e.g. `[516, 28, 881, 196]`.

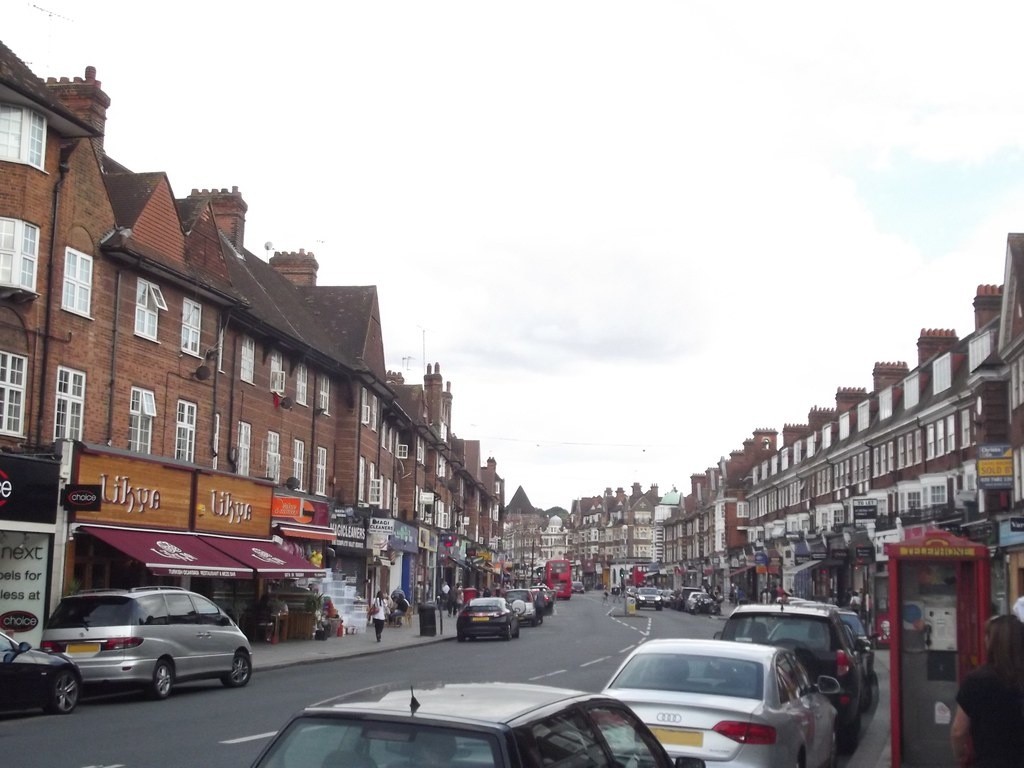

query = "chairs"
[746, 622, 768, 644]
[252, 611, 270, 642]
[320, 728, 379, 768]
[668, 657, 690, 689]
[409, 732, 460, 768]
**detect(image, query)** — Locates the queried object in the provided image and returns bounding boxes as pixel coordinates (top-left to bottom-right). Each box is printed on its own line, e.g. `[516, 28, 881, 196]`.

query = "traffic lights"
[620, 569, 624, 577]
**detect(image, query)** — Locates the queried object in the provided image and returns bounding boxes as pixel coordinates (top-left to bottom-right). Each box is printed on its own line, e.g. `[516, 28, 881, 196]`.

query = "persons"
[387, 594, 408, 625]
[1012, 596, 1024, 624]
[949, 613, 1024, 768]
[858, 588, 864, 602]
[743, 621, 773, 646]
[474, 584, 481, 598]
[397, 593, 410, 626]
[759, 583, 788, 604]
[482, 584, 491, 597]
[252, 594, 274, 642]
[842, 586, 853, 609]
[371, 591, 388, 642]
[441, 581, 464, 617]
[847, 590, 861, 614]
[705, 582, 745, 609]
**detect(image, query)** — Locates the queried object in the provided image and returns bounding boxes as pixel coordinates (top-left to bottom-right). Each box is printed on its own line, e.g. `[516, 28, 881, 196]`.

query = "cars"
[531, 585, 556, 615]
[0, 630, 88, 716]
[457, 596, 521, 642]
[243, 679, 709, 768]
[657, 586, 720, 616]
[627, 587, 638, 598]
[774, 597, 877, 711]
[595, 637, 844, 768]
[635, 587, 663, 612]
[572, 581, 585, 592]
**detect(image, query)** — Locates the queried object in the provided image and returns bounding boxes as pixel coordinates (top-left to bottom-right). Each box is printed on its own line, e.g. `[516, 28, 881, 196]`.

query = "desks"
[269, 614, 288, 643]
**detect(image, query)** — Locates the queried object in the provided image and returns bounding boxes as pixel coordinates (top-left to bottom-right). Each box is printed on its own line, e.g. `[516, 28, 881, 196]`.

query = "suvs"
[39, 585, 255, 701]
[504, 589, 539, 627]
[710, 602, 871, 755]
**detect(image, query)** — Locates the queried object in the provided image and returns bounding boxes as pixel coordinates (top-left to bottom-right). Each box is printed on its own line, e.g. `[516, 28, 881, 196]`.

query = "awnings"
[81, 523, 328, 579]
[275, 521, 337, 541]
[723, 566, 755, 579]
[788, 559, 825, 575]
[449, 556, 501, 576]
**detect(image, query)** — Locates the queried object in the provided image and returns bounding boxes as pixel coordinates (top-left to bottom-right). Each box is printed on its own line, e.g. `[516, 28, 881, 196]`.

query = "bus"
[544, 558, 572, 600]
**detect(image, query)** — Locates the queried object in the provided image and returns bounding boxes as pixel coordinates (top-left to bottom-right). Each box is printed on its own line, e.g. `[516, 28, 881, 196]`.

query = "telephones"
[925, 608, 959, 652]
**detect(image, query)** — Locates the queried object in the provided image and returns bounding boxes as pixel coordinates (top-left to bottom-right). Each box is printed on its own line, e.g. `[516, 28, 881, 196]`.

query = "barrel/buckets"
[346, 626, 355, 635]
[462, 587, 478, 604]
[626, 596, 635, 614]
[419, 603, 437, 637]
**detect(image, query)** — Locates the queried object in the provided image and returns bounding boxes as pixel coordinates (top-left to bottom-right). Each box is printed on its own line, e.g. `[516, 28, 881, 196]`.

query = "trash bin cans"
[417, 603, 436, 636]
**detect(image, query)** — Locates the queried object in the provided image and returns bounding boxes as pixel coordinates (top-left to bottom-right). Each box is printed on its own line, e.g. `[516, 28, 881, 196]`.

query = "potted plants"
[305, 595, 329, 641]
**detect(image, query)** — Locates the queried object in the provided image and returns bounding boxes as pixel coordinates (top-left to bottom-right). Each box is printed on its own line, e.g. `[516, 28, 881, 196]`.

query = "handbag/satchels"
[368, 607, 379, 615]
